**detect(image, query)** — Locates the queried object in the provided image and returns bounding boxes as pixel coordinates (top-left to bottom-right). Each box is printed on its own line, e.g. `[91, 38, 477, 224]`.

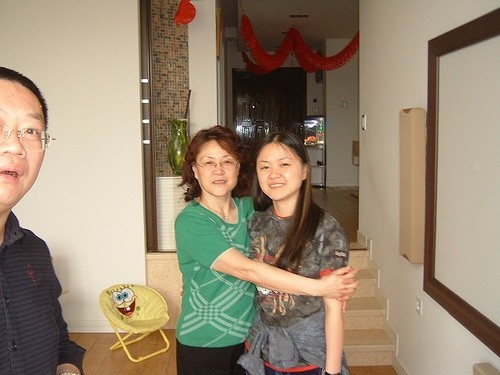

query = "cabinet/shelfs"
[305, 146, 325, 187]
[156, 175, 193, 252]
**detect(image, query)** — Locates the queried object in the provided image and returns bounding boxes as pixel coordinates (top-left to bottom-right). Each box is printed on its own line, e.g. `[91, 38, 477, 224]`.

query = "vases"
[169, 117, 189, 176]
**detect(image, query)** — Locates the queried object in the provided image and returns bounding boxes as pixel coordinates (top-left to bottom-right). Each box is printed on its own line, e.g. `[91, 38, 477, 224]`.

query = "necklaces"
[271, 207, 287, 218]
[203, 201, 231, 222]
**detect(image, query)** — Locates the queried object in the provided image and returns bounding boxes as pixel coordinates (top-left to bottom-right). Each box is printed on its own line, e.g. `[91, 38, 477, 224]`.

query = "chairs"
[99, 283, 170, 363]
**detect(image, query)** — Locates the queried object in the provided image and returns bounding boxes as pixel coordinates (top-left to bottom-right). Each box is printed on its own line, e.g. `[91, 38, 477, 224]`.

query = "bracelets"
[322, 368, 342, 375]
[60, 372, 80, 375]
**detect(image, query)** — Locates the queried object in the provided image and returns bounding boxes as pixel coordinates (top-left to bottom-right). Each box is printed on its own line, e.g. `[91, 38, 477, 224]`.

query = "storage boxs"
[303, 115, 325, 147]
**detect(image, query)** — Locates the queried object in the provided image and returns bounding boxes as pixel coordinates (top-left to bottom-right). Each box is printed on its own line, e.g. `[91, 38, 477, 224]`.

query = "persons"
[0, 67, 87, 375]
[237, 133, 352, 375]
[175, 124, 360, 375]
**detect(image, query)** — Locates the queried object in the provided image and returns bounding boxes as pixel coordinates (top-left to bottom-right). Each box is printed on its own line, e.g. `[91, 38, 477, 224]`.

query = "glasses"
[0, 122, 56, 149]
[196, 158, 239, 170]
